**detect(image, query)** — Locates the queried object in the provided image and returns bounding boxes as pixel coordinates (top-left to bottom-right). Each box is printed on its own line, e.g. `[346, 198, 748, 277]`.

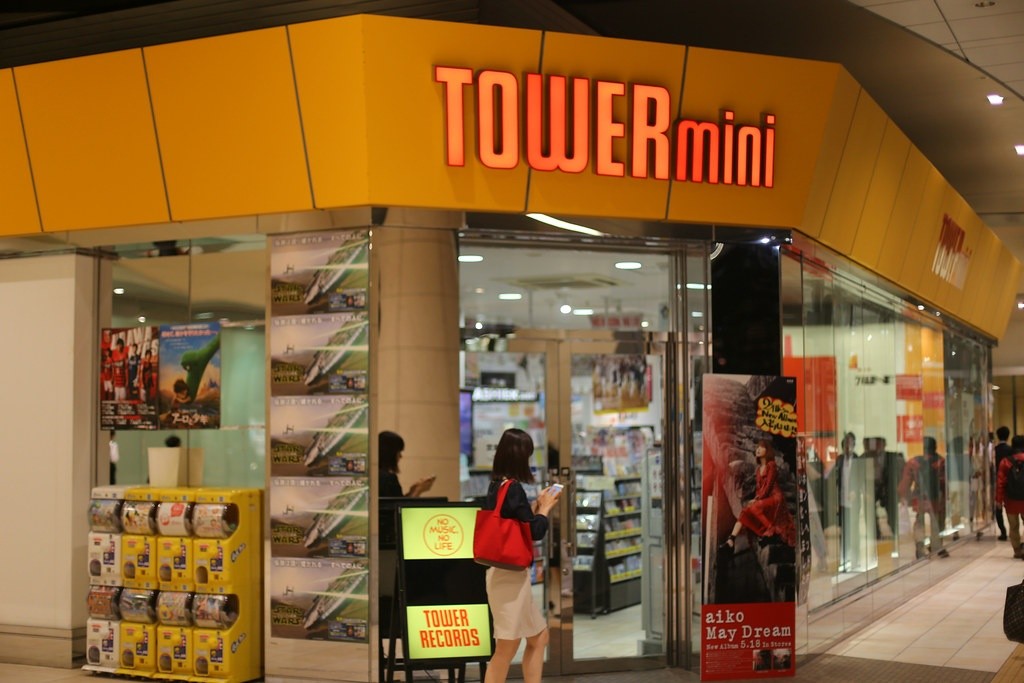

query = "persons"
[102, 337, 155, 419]
[801, 430, 988, 560]
[718, 439, 797, 557]
[147, 436, 183, 485]
[379, 429, 437, 671]
[996, 435, 1024, 560]
[989, 432, 996, 521]
[109, 429, 119, 485]
[991, 425, 1014, 540]
[482, 427, 563, 683]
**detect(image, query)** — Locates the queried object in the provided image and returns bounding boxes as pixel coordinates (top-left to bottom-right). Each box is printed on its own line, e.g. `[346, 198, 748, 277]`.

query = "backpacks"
[915, 455, 941, 500]
[1006, 455, 1024, 500]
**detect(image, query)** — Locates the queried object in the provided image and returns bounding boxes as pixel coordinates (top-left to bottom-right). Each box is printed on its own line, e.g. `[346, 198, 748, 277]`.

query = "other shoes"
[876, 526, 981, 559]
[719, 534, 736, 553]
[758, 531, 779, 546]
[998, 535, 1024, 560]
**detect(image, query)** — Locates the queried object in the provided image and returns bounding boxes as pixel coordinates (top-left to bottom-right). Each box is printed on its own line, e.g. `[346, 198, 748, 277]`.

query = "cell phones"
[548, 483, 564, 498]
[424, 475, 438, 483]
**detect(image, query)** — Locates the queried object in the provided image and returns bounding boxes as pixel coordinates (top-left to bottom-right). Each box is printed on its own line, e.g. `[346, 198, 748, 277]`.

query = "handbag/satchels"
[1003, 578, 1024, 645]
[473, 480, 534, 571]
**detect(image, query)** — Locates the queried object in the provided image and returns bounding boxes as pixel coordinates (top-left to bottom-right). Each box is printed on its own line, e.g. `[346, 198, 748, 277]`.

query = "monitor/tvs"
[400, 506, 496, 661]
